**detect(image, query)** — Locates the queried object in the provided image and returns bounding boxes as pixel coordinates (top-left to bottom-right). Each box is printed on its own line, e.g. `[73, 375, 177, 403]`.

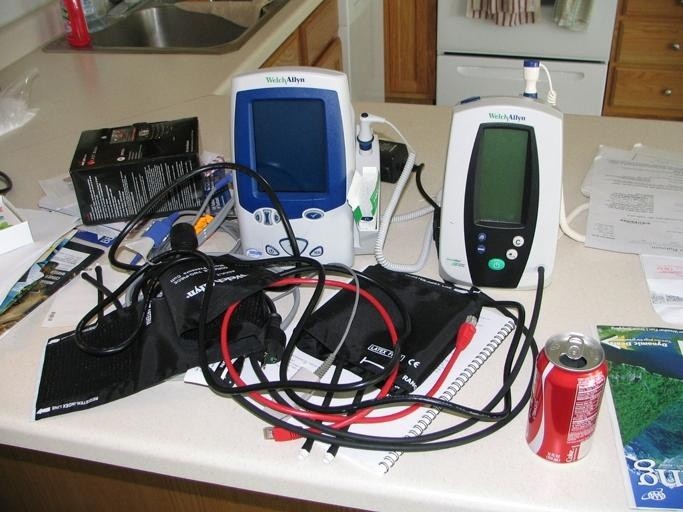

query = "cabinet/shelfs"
[385, 0, 437, 104]
[602, 0, 683, 120]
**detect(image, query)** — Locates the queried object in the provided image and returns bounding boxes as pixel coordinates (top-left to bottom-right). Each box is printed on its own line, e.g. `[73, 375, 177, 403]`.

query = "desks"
[0, 1, 682, 509]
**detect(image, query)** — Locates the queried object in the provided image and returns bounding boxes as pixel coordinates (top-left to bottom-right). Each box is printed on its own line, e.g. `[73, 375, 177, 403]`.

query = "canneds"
[525, 331, 608, 464]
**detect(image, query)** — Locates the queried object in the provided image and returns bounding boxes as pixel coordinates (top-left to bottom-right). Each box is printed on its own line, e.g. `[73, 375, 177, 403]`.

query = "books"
[264, 277, 520, 475]
